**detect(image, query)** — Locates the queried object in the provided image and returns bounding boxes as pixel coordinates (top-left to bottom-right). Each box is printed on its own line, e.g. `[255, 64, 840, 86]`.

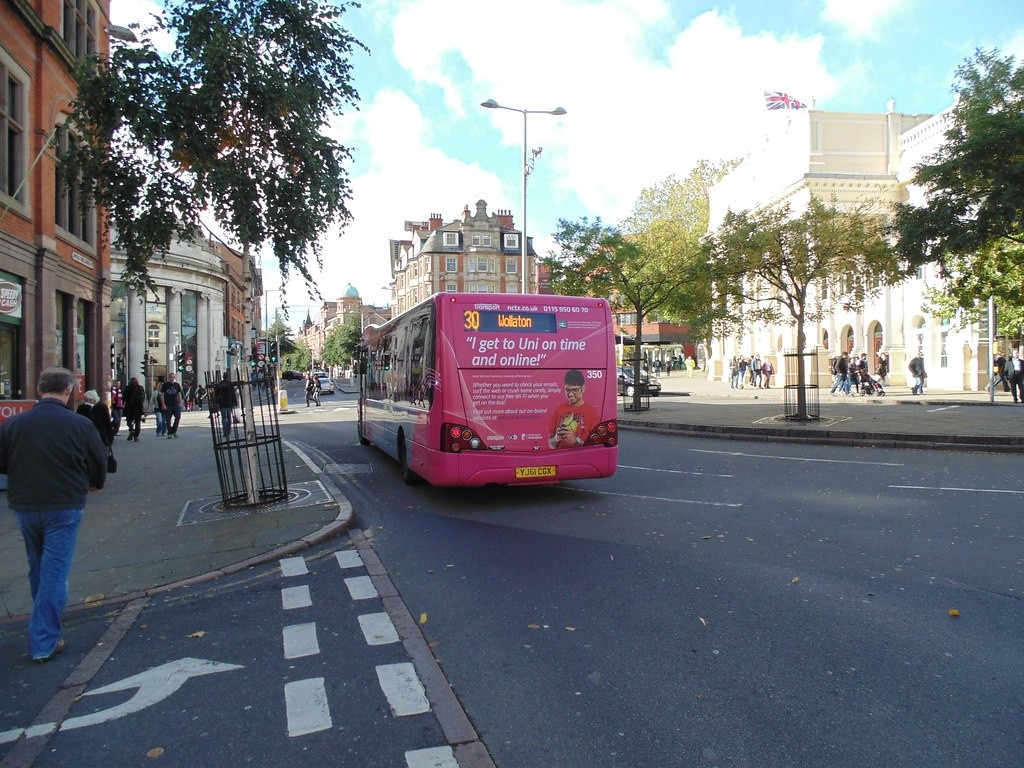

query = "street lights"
[265, 286, 286, 369]
[481, 97, 568, 295]
[275, 304, 291, 378]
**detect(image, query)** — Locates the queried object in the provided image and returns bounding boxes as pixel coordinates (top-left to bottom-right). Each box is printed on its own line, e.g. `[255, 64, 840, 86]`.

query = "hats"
[861, 353, 868, 358]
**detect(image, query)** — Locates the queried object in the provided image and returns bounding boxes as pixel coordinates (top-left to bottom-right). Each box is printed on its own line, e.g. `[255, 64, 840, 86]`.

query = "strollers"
[854, 367, 885, 398]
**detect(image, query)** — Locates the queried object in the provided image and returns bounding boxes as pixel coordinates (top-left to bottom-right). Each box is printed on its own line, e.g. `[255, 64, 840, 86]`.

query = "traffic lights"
[251, 347, 258, 369]
[287, 358, 291, 367]
[270, 341, 278, 363]
[140, 358, 149, 378]
[110, 341, 115, 370]
[177, 350, 186, 373]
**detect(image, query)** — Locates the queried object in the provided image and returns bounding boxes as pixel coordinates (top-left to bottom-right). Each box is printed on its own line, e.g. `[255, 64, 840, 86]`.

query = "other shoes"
[163, 432, 166, 436]
[134, 435, 139, 443]
[174, 433, 179, 438]
[127, 429, 135, 440]
[36, 638, 64, 662]
[985, 387, 990, 392]
[168, 433, 174, 439]
[911, 387, 914, 393]
[921, 392, 926, 395]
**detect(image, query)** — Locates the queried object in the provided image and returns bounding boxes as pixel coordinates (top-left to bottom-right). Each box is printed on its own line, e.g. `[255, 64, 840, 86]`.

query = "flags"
[762, 90, 808, 110]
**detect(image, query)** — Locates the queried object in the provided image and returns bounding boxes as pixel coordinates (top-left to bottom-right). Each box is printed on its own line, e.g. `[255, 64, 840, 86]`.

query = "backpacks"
[829, 360, 837, 375]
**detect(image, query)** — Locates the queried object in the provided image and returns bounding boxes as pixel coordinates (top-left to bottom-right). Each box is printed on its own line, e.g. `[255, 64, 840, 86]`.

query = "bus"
[357, 291, 620, 492]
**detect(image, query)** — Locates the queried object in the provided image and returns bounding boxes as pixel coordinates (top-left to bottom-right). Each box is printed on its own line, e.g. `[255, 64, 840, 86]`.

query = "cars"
[281, 370, 303, 380]
[315, 370, 335, 394]
[616, 366, 662, 397]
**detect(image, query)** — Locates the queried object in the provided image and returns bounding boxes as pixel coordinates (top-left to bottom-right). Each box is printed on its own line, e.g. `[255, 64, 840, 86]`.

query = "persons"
[677, 355, 682, 370]
[692, 354, 698, 368]
[728, 354, 773, 390]
[671, 355, 676, 370]
[653, 358, 663, 377]
[830, 351, 887, 396]
[703, 358, 706, 371]
[304, 375, 321, 407]
[643, 363, 649, 375]
[548, 370, 600, 448]
[122, 378, 145, 442]
[201, 382, 220, 419]
[161, 373, 182, 438]
[665, 356, 673, 375]
[77, 389, 113, 492]
[251, 369, 274, 390]
[111, 381, 122, 436]
[179, 383, 208, 411]
[985, 349, 1024, 403]
[214, 372, 237, 437]
[151, 383, 169, 436]
[909, 352, 927, 394]
[0, 367, 107, 665]
[684, 356, 695, 378]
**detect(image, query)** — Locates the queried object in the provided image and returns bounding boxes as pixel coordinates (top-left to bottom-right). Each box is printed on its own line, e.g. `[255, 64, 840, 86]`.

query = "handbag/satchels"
[107, 447, 117, 473]
